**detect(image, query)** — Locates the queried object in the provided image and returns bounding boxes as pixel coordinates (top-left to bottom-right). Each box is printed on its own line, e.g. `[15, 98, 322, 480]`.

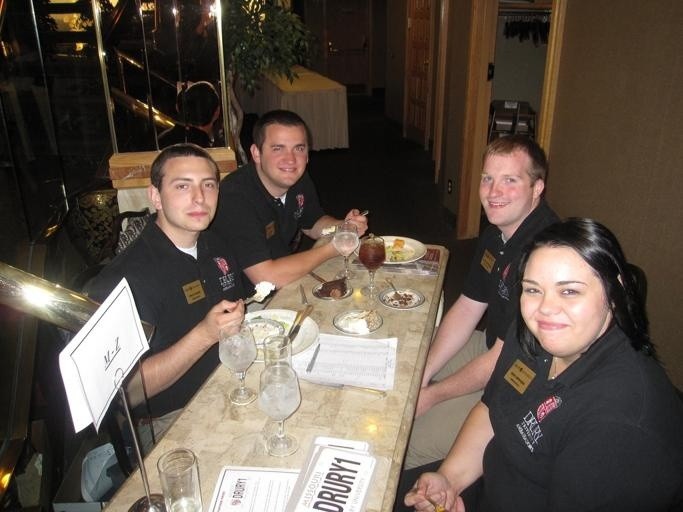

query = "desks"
[239, 56, 349, 155]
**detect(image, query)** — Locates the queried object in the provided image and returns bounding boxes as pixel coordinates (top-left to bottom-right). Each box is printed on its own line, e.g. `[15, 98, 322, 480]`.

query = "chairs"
[44, 255, 182, 478]
[100, 208, 154, 261]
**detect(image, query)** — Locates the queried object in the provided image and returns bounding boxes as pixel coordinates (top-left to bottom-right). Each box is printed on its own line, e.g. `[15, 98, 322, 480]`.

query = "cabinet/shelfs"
[486, 100, 537, 146]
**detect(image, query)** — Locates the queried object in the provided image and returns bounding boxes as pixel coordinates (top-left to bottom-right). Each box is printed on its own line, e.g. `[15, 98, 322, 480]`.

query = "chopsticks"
[305, 343, 320, 373]
[309, 271, 329, 286]
[299, 283, 309, 304]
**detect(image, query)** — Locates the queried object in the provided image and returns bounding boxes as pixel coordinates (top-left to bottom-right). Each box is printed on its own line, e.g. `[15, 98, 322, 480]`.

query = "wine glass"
[216, 324, 258, 406]
[357, 235, 386, 297]
[332, 224, 359, 282]
[257, 365, 301, 459]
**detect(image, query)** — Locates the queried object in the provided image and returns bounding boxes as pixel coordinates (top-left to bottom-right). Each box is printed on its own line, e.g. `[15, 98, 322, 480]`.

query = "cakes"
[318, 276, 348, 299]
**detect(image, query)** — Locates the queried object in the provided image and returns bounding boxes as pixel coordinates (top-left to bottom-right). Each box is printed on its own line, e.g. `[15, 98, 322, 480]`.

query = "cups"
[156, 447, 202, 512]
[262, 335, 292, 366]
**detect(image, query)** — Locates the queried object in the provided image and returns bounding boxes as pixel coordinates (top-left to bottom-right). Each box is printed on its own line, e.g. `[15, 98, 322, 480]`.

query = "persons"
[390, 215, 681, 509]
[197, 109, 368, 291]
[402, 134, 564, 470]
[83, 143, 245, 458]
[155, 81, 220, 150]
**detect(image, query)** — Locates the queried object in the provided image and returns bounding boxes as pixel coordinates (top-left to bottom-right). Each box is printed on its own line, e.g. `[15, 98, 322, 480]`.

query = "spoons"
[385, 276, 402, 299]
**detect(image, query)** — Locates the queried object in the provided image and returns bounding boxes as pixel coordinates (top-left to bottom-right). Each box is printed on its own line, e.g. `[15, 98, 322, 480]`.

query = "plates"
[377, 288, 425, 309]
[332, 309, 383, 336]
[310, 282, 353, 300]
[243, 309, 321, 363]
[378, 235, 427, 266]
[238, 318, 285, 346]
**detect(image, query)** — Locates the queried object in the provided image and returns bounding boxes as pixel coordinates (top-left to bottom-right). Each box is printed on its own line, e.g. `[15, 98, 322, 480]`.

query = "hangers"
[500, 9, 550, 49]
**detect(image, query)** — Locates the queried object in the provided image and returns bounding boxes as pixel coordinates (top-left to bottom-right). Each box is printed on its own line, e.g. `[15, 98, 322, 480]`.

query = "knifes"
[302, 376, 387, 399]
[288, 305, 313, 344]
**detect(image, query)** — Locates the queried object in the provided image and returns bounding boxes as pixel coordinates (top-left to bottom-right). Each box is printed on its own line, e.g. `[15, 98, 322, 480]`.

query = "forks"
[238, 293, 264, 306]
[321, 210, 368, 235]
